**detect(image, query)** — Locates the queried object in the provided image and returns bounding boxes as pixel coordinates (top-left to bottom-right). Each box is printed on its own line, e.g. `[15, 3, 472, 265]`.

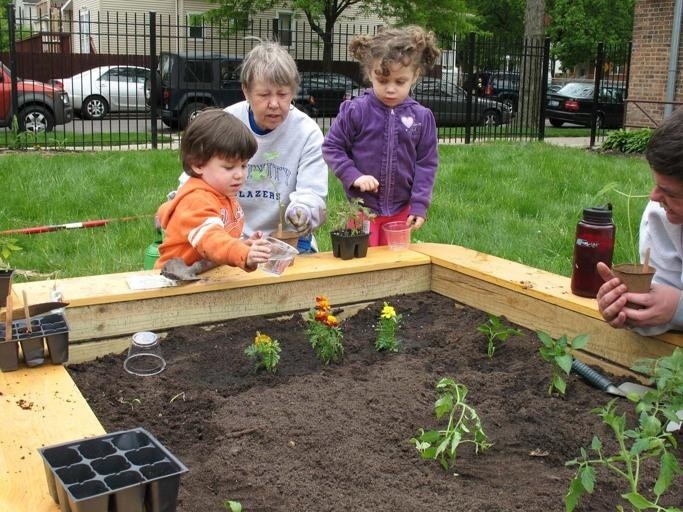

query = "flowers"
[243, 294, 403, 378]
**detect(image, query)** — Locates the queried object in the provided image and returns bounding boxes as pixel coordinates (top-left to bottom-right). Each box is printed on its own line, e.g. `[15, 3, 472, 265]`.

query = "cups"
[382, 220, 414, 252]
[256, 237, 300, 276]
[123, 331, 167, 377]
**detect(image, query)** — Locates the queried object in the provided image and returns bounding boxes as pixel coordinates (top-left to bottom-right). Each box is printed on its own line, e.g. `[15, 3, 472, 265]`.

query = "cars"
[46, 63, 152, 120]
[296, 69, 364, 118]
[342, 76, 513, 128]
[0, 61, 72, 134]
[544, 82, 626, 130]
[460, 70, 564, 115]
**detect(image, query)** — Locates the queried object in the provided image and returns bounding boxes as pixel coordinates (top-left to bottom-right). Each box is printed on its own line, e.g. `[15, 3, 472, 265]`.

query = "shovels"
[571, 358, 669, 403]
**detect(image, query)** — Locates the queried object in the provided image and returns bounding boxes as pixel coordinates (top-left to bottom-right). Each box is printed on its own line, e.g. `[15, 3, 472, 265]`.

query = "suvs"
[143, 50, 318, 131]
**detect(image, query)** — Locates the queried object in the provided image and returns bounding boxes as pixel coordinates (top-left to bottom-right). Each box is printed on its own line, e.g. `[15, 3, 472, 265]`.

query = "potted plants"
[325, 197, 376, 263]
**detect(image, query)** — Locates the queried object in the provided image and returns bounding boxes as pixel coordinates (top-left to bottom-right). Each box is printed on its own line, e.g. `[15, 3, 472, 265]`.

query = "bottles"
[570, 202, 617, 299]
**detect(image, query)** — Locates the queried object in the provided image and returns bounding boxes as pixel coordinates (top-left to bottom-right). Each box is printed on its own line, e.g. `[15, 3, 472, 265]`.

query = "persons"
[596, 105, 683, 336]
[153, 106, 271, 272]
[319, 27, 444, 246]
[169, 38, 328, 255]
